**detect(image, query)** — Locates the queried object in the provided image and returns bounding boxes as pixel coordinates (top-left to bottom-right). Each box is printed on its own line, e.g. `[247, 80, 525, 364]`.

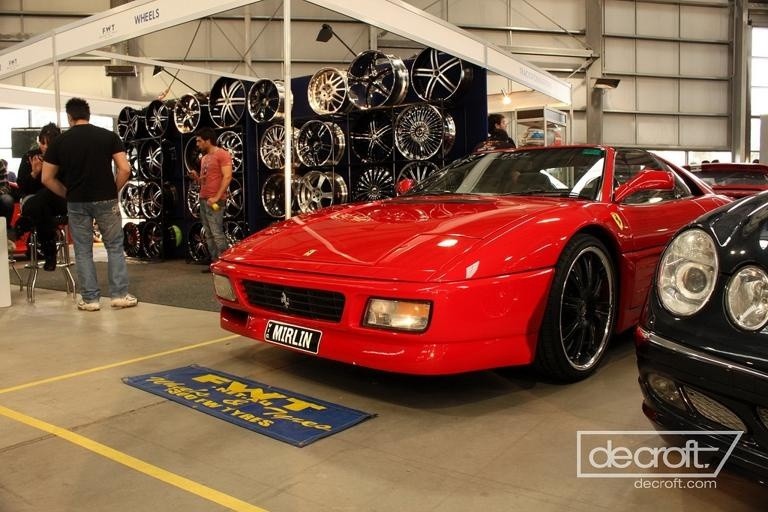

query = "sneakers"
[76, 299, 101, 312]
[109, 293, 139, 308]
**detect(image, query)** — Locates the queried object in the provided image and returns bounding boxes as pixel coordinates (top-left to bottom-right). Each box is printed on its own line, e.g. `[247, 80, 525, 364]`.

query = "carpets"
[122, 363, 372, 450]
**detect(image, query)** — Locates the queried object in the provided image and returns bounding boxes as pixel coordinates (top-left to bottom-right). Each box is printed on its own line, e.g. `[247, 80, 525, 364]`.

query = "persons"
[0, 158, 23, 242]
[188, 128, 232, 273]
[7, 123, 68, 272]
[690, 159, 719, 170]
[477, 114, 518, 193]
[40, 97, 138, 313]
[752, 159, 759, 163]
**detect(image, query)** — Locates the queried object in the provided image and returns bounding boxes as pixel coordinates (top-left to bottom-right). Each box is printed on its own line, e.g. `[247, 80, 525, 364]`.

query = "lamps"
[150, 67, 206, 101]
[314, 23, 359, 57]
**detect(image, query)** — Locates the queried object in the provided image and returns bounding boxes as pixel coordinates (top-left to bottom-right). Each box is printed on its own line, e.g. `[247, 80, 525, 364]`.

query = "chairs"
[515, 173, 554, 191]
